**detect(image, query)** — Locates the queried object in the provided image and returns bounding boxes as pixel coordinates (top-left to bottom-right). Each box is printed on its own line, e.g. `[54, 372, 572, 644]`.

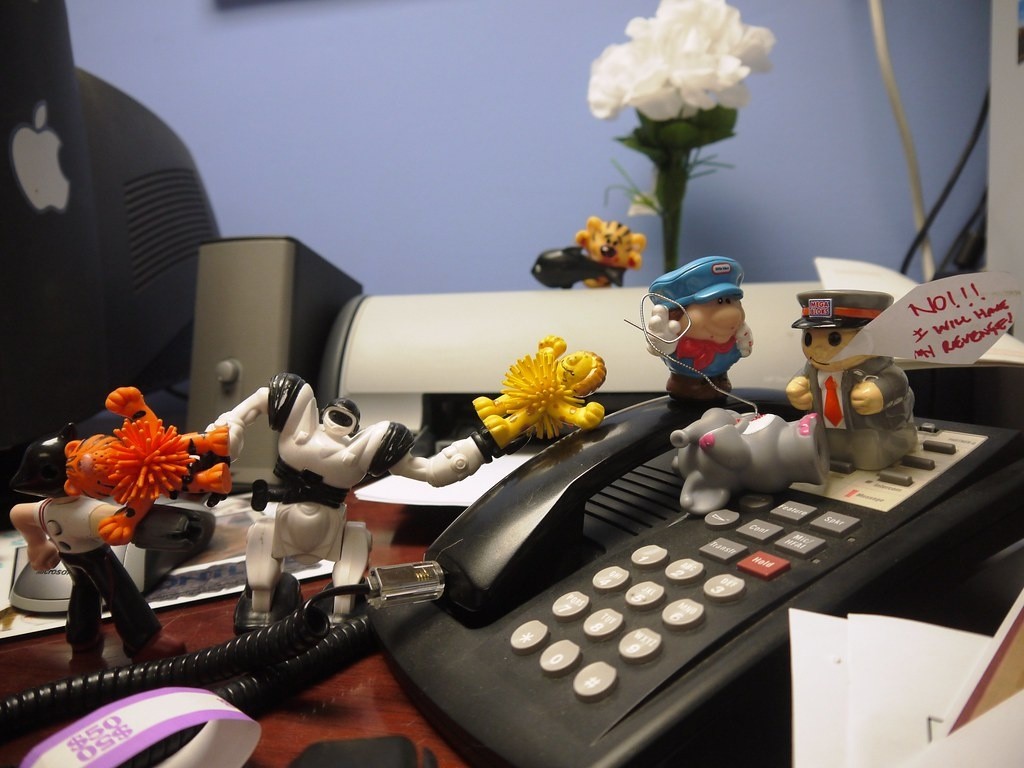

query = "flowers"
[587, 0, 775, 273]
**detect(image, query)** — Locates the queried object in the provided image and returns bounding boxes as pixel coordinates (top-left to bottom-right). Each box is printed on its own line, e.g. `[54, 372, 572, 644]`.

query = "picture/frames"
[0, 482, 349, 639]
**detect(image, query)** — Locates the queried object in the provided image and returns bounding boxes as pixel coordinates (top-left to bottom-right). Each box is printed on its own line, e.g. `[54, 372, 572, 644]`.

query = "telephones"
[366, 390, 1024, 768]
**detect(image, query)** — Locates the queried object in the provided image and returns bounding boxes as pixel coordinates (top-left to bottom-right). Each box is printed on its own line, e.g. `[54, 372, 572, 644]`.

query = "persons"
[786, 289, 921, 471]
[11, 426, 185, 674]
[647, 255, 754, 405]
[208, 373, 531, 630]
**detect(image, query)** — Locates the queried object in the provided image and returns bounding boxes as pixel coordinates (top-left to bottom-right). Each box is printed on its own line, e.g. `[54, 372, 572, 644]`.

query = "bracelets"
[20, 687, 261, 767]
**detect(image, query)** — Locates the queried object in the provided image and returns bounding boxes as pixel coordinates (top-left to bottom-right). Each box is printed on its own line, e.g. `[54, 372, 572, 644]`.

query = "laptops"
[0, 0, 111, 445]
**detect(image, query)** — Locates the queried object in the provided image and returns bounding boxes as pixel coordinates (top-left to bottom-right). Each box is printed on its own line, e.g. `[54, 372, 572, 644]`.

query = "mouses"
[9, 501, 218, 616]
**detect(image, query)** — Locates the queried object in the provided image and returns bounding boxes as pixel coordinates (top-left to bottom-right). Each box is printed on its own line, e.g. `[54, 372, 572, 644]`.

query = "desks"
[0, 484, 477, 768]
